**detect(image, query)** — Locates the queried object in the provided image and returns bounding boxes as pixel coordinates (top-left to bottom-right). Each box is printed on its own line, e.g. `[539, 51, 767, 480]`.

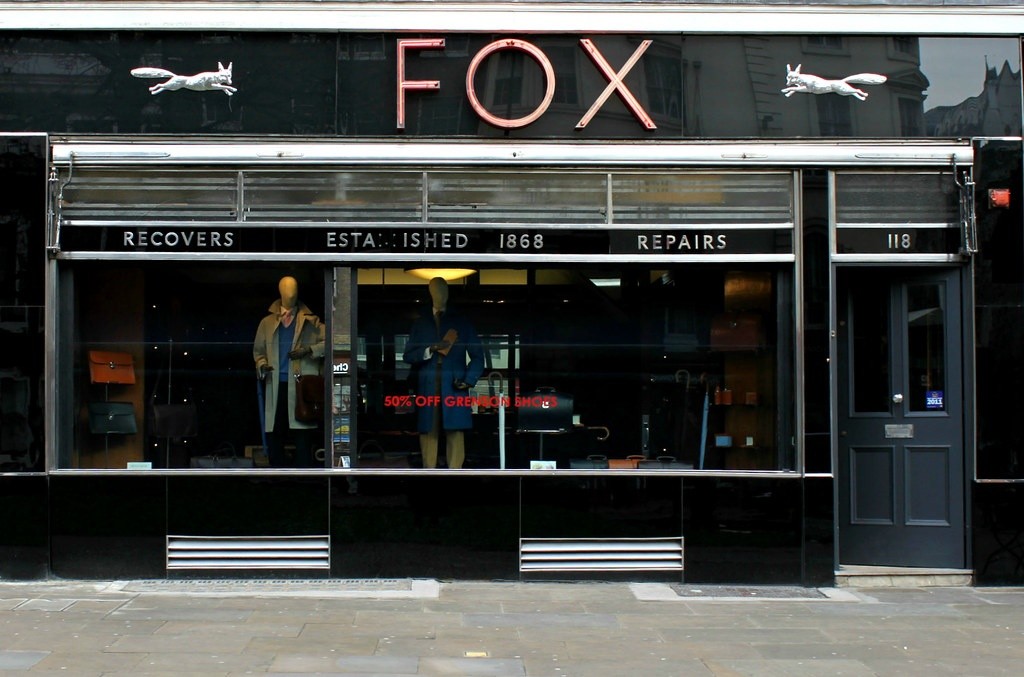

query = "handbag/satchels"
[637, 456, 693, 469]
[569, 455, 609, 469]
[295, 374, 325, 422]
[89, 351, 136, 385]
[150, 403, 197, 439]
[607, 455, 660, 468]
[89, 401, 138, 435]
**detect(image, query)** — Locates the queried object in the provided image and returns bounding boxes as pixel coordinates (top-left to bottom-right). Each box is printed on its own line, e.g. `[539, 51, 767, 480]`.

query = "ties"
[285, 311, 292, 326]
[436, 311, 442, 337]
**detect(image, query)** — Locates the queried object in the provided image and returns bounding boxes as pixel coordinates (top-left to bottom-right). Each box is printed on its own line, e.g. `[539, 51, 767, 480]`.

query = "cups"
[722, 390, 732, 405]
[715, 391, 722, 405]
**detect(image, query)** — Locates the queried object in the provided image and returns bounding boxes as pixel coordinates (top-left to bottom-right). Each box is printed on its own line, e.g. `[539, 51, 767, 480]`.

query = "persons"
[253, 276, 325, 468]
[403, 277, 485, 469]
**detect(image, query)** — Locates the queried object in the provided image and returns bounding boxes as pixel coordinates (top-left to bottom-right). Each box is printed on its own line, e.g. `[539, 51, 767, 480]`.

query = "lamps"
[588, 270, 622, 286]
[975, 188, 1011, 208]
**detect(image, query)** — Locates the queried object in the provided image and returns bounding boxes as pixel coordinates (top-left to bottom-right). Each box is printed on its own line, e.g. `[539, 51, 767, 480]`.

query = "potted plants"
[404, 265, 478, 279]
